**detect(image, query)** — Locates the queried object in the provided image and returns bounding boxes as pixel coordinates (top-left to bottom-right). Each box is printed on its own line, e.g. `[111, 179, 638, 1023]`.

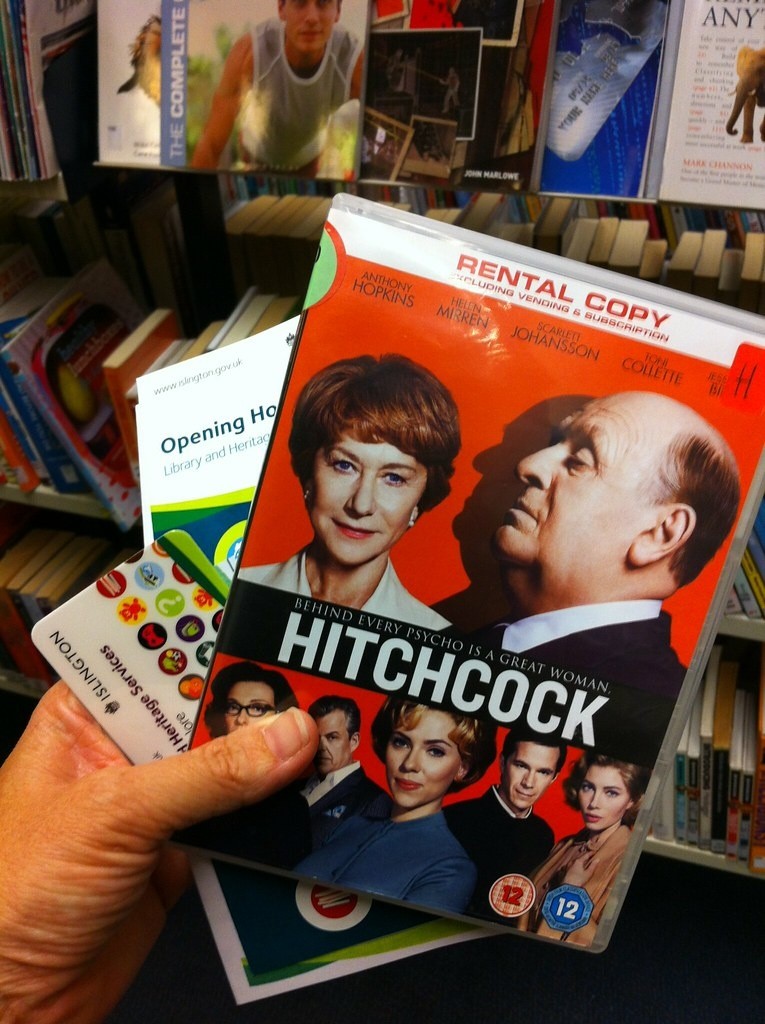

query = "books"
[1, 0, 764, 208]
[153, 191, 763, 958]
[0, 180, 763, 877]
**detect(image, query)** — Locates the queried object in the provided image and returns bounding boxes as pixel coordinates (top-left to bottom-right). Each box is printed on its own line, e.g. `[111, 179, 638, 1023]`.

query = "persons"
[266, 694, 395, 862]
[1, 680, 318, 1024]
[442, 726, 569, 926]
[470, 391, 741, 765]
[513, 750, 655, 948]
[287, 690, 497, 915]
[177, 660, 312, 871]
[234, 353, 462, 632]
[187, 1, 366, 179]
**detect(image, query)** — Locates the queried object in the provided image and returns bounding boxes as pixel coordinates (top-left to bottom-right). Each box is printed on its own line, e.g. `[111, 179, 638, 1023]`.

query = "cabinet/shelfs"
[0, 482, 765, 880]
